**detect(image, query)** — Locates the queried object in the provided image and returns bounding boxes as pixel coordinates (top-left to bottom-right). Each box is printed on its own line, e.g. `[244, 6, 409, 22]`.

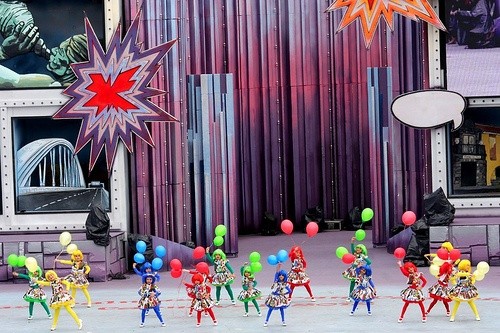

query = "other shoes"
[264, 321, 268, 327]
[425, 312, 429, 316]
[79, 319, 83, 330]
[350, 310, 355, 316]
[287, 298, 292, 303]
[422, 316, 427, 322]
[51, 328, 55, 330]
[446, 312, 450, 317]
[450, 319, 455, 322]
[48, 314, 52, 319]
[310, 296, 316, 301]
[87, 304, 92, 308]
[398, 316, 404, 323]
[146, 310, 150, 315]
[160, 322, 166, 326]
[346, 297, 351, 302]
[71, 304, 76, 308]
[282, 321, 286, 326]
[367, 310, 372, 316]
[476, 317, 481, 320]
[189, 300, 262, 328]
[28, 316, 32, 320]
[140, 323, 144, 327]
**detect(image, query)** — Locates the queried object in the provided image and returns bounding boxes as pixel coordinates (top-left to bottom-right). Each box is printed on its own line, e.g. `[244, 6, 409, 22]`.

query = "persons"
[237, 261, 263, 317]
[12, 265, 52, 318]
[133, 263, 166, 328]
[288, 245, 317, 302]
[424, 240, 461, 278]
[181, 249, 236, 328]
[397, 260, 481, 323]
[54, 249, 92, 309]
[32, 270, 83, 332]
[263, 263, 292, 326]
[342, 237, 376, 315]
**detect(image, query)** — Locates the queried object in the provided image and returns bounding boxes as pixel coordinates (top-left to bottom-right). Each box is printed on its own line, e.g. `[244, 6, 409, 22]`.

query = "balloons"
[334, 230, 366, 264]
[7, 253, 38, 271]
[436, 248, 490, 281]
[392, 247, 407, 261]
[170, 246, 208, 278]
[360, 207, 374, 223]
[213, 224, 228, 246]
[306, 222, 318, 237]
[248, 248, 288, 272]
[281, 220, 293, 235]
[132, 241, 166, 270]
[402, 211, 416, 226]
[60, 231, 77, 254]
